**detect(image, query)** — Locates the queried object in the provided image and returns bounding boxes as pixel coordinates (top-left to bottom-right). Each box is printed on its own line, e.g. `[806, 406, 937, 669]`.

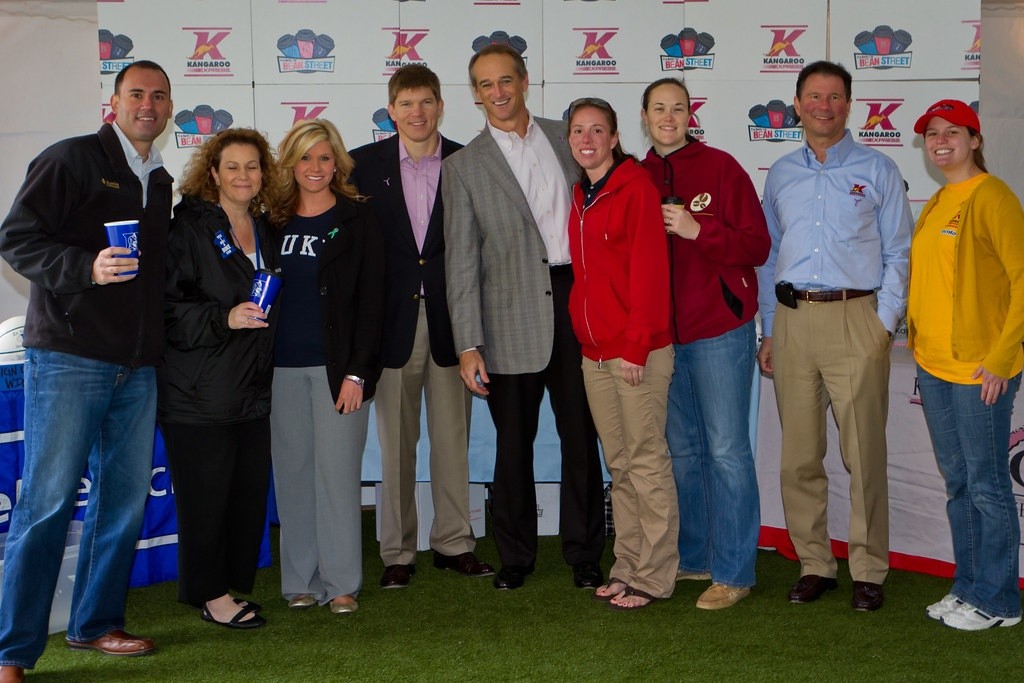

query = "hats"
[915, 98, 981, 132]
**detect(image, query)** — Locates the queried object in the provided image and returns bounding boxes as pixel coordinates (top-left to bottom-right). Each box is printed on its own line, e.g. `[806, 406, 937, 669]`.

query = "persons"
[157, 129, 281, 629]
[441, 46, 605, 593]
[907, 100, 1024, 631]
[256, 119, 388, 613]
[568, 96, 680, 609]
[640, 78, 772, 611]
[345, 64, 495, 590]
[754, 61, 915, 612]
[0, 60, 174, 683]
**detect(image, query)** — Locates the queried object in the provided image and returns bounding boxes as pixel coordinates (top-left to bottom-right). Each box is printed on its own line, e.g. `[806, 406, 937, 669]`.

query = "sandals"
[201, 602, 267, 629]
[234, 598, 263, 613]
[608, 586, 658, 610]
[593, 577, 630, 601]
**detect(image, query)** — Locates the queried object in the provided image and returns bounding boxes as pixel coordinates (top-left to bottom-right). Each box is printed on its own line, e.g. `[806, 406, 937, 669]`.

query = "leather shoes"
[852, 581, 884, 611]
[572, 567, 604, 591]
[380, 564, 415, 589]
[494, 565, 526, 591]
[68, 628, 158, 658]
[788, 574, 840, 604]
[0, 666, 24, 683]
[432, 550, 496, 576]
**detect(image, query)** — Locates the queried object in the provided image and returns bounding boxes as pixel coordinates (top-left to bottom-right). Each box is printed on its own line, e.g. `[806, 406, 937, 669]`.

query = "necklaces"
[219, 203, 242, 251]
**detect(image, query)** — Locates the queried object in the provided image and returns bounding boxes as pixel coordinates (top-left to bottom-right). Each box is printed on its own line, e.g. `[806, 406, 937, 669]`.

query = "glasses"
[567, 98, 613, 121]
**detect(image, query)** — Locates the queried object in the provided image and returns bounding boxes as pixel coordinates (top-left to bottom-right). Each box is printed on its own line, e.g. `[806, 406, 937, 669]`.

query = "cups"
[104, 220, 140, 276]
[249, 269, 283, 323]
[662, 197, 685, 233]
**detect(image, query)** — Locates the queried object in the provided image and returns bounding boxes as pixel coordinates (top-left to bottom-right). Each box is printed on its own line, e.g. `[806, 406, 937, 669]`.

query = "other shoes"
[675, 568, 712, 580]
[330, 597, 358, 614]
[697, 582, 751, 610]
[288, 595, 315, 608]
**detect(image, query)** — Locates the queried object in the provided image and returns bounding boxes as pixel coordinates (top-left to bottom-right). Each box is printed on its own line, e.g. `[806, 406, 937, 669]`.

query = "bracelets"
[346, 375, 364, 384]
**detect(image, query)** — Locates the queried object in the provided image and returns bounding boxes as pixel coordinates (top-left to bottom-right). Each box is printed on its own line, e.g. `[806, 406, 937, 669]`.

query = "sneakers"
[925, 593, 964, 623]
[941, 602, 1022, 631]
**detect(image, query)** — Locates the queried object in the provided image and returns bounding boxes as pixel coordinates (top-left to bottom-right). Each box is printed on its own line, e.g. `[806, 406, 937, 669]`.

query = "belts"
[793, 289, 873, 301]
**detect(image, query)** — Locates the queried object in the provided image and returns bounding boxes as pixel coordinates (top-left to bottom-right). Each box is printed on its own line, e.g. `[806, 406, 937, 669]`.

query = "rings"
[245, 318, 249, 324]
[668, 219, 672, 225]
